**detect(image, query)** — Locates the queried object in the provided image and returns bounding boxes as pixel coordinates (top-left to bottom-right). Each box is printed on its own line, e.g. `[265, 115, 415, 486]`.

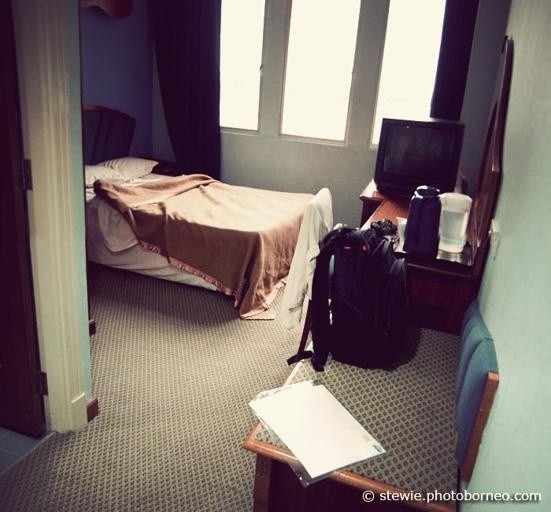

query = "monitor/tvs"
[373, 117, 465, 195]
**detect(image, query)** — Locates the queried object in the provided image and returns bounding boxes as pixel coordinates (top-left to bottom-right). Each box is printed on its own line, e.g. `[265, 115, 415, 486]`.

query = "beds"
[82, 104, 315, 320]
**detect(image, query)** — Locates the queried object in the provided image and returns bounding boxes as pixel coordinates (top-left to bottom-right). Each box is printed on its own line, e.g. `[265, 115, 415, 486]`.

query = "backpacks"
[286, 225, 422, 372]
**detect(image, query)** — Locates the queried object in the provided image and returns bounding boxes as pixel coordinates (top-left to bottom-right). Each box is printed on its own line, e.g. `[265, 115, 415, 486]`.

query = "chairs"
[282, 187, 333, 353]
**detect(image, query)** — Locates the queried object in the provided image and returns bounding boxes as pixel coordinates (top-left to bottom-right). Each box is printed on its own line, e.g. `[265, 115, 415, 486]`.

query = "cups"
[439, 192, 473, 253]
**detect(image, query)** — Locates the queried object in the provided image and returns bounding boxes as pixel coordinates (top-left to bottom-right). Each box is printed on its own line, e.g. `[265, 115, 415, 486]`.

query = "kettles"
[402, 185, 442, 259]
